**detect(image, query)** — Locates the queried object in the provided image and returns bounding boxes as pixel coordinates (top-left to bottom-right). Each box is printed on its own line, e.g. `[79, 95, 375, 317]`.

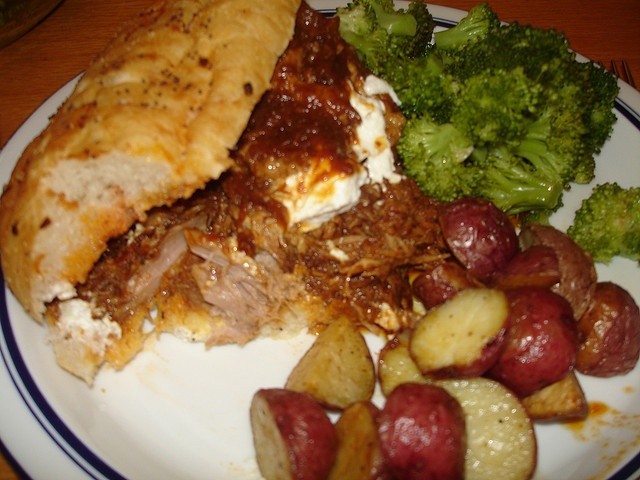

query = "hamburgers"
[0, 1, 301, 323]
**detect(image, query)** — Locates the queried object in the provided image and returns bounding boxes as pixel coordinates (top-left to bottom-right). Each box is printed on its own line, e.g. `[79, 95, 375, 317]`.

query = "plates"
[0, 0, 640, 479]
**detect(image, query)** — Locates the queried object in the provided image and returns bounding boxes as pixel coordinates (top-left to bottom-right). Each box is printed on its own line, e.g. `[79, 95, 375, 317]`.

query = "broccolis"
[336, 0, 640, 265]
